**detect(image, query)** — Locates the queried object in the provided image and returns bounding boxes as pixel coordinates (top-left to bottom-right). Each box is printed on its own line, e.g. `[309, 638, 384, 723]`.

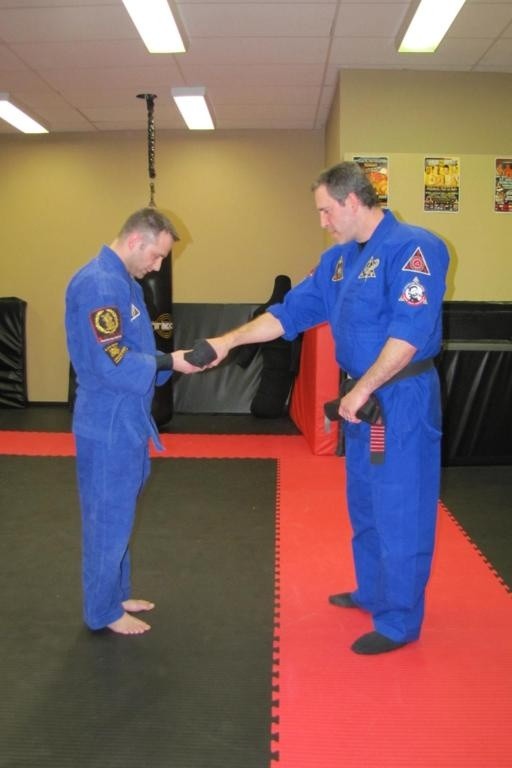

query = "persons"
[425, 164, 459, 212]
[65, 208, 208, 639]
[193, 159, 452, 657]
[495, 160, 512, 211]
